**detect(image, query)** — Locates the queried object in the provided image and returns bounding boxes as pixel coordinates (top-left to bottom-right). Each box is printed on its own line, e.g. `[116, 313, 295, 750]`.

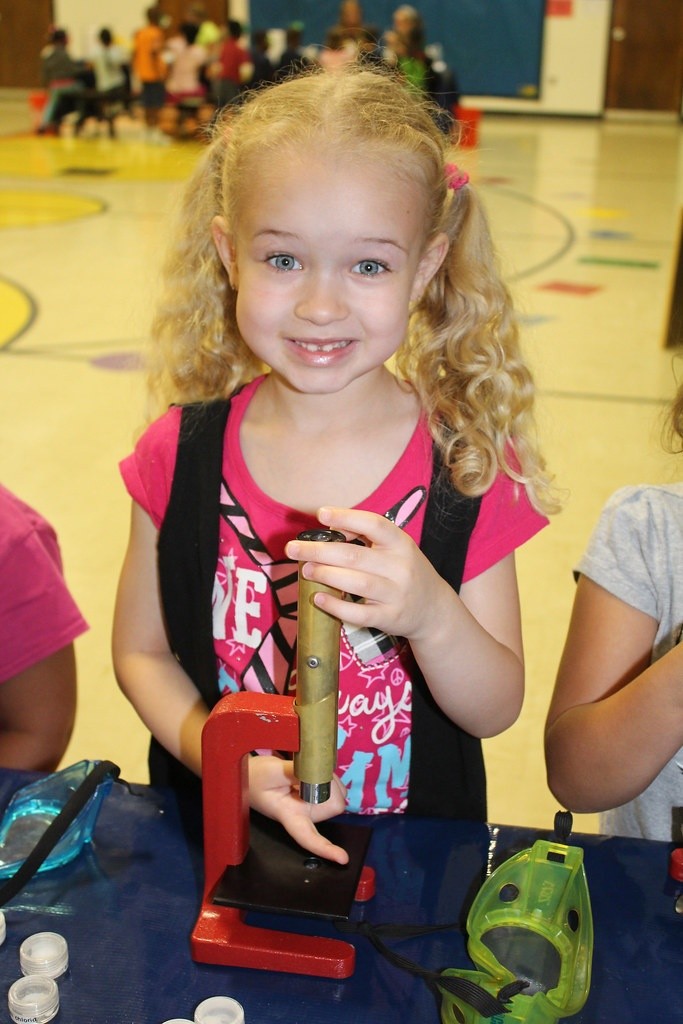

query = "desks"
[0, 768, 682, 1024]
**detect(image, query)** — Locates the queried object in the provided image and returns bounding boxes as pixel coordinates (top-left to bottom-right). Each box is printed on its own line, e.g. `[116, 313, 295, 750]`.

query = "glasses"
[339, 806, 595, 1024]
[0, 759, 121, 905]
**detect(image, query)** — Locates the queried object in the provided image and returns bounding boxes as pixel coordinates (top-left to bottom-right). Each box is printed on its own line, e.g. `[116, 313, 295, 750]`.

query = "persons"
[111, 64, 525, 866]
[0, 485, 90, 769]
[545, 379, 683, 841]
[37, 0, 453, 136]
